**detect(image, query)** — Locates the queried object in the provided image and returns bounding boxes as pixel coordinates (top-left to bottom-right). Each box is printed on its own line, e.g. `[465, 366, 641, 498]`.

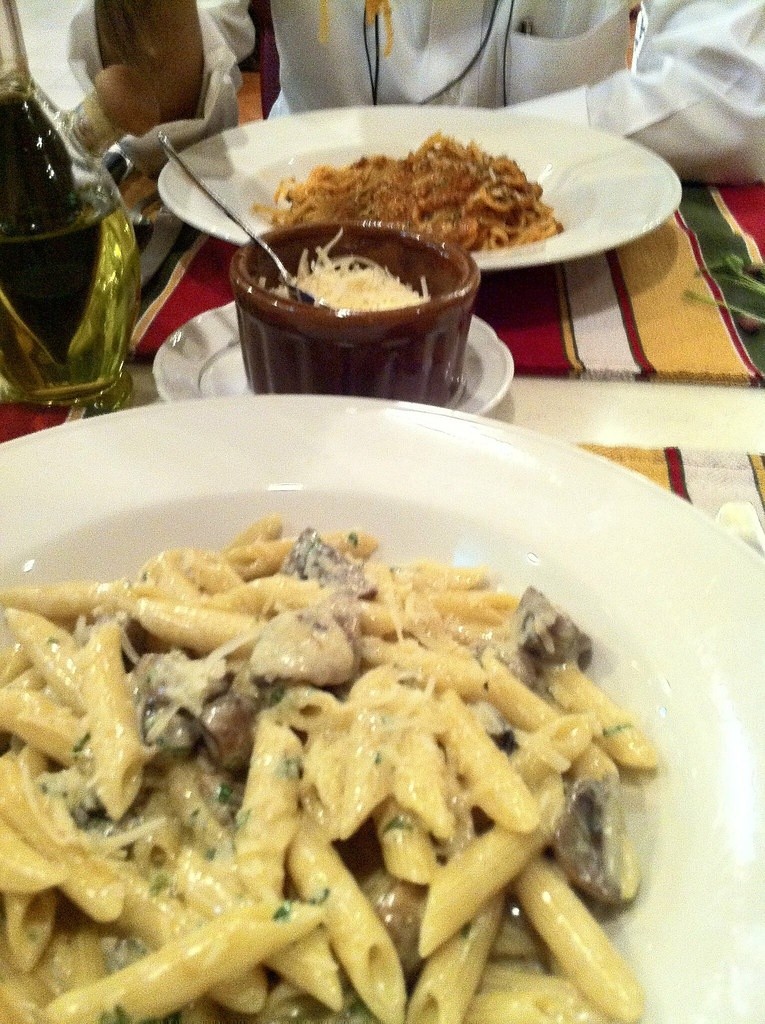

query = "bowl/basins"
[230, 217, 477, 408]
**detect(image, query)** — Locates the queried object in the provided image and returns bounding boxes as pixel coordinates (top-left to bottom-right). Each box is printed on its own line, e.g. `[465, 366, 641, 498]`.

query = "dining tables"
[0, 170, 765, 1024]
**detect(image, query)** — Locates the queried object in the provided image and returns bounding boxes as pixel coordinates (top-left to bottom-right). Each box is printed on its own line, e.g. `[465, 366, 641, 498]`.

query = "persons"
[66, 0, 765, 186]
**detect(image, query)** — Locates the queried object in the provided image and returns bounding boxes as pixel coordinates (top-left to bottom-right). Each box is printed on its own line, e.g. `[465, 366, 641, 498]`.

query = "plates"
[8, 394, 765, 1024]
[158, 102, 683, 270]
[151, 301, 514, 415]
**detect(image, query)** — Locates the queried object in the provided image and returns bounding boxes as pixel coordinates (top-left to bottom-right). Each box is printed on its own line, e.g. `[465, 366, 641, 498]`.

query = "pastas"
[0, 518, 659, 1024]
[254, 134, 561, 255]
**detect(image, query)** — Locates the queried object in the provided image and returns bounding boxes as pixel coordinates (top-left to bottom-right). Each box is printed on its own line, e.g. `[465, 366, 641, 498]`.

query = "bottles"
[2, 1, 132, 413]
